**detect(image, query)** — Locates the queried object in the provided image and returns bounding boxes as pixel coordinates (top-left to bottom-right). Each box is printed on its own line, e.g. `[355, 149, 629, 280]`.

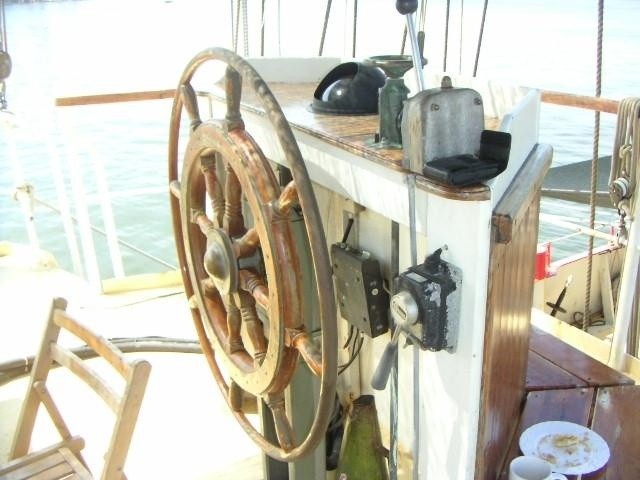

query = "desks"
[499, 308, 640, 479]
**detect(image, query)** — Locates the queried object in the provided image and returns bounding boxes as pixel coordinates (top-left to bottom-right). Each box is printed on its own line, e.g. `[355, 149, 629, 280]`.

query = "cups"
[508, 456, 568, 480]
[535, 246, 548, 280]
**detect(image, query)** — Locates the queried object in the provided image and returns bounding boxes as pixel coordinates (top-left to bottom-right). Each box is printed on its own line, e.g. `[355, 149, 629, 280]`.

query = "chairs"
[0, 296, 152, 480]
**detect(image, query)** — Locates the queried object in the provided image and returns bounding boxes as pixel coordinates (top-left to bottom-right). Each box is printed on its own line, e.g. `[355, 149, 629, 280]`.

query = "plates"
[518, 420, 611, 475]
